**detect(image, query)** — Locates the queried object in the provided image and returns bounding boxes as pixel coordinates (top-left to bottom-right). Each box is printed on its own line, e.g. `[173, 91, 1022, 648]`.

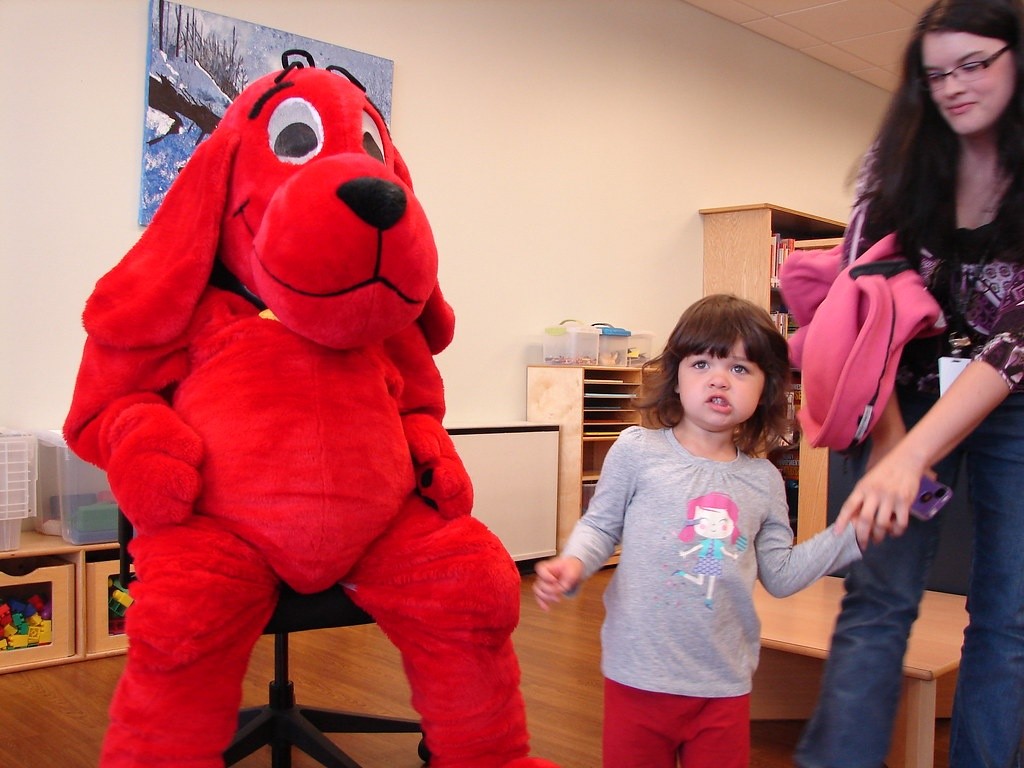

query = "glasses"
[917, 44, 1011, 91]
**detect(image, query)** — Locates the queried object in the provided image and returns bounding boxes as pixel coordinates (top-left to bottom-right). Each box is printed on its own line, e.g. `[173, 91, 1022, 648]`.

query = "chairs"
[112, 502, 432, 768]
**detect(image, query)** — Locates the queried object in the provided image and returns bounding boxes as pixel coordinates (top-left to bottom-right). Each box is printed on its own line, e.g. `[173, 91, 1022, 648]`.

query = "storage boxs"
[589, 322, 631, 367]
[768, 450, 800, 480]
[543, 319, 599, 366]
[626, 328, 656, 369]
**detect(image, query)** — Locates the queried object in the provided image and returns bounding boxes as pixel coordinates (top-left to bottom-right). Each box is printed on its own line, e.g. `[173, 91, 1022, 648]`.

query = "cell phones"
[909, 476, 953, 522]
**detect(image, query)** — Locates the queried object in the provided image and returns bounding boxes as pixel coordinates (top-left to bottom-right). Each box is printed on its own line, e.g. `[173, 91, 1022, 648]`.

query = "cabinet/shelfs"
[0, 529, 139, 674]
[699, 203, 851, 548]
[525, 365, 663, 567]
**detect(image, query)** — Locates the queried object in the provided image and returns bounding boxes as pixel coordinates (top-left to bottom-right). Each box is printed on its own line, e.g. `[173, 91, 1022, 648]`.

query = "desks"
[750, 574, 969, 768]
[441, 421, 560, 573]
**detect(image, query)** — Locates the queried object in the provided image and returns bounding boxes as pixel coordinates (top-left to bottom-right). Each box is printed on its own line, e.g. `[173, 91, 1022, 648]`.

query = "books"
[770, 305, 799, 341]
[770, 450, 799, 488]
[771, 231, 795, 289]
[766, 378, 802, 446]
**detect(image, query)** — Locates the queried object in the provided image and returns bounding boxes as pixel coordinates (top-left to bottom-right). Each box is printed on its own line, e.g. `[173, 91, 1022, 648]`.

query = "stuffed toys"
[62, 49, 562, 768]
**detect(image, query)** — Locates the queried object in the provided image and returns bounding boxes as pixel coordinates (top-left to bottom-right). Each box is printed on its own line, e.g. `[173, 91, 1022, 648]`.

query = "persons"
[793, 0, 1024, 768]
[533, 293, 897, 768]
